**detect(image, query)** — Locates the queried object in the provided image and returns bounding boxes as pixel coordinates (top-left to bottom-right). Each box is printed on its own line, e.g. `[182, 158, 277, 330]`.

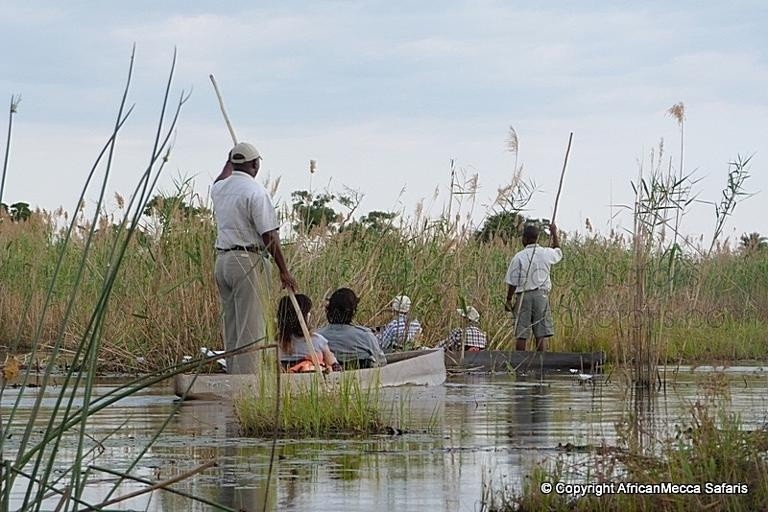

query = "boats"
[172, 348, 447, 401]
[444, 349, 605, 372]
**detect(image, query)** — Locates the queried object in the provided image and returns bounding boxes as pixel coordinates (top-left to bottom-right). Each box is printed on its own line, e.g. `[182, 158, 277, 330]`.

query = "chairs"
[280, 356, 371, 374]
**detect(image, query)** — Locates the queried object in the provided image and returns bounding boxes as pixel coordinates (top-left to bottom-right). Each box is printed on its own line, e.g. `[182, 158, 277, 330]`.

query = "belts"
[216, 245, 269, 259]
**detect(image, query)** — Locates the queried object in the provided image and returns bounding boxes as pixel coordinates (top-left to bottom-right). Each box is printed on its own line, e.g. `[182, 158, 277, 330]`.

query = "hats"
[329, 288, 361, 307]
[456, 306, 480, 323]
[392, 294, 412, 313]
[230, 143, 263, 163]
[524, 226, 539, 238]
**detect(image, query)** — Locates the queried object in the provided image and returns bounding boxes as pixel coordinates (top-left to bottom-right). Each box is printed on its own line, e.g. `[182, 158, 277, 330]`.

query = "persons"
[434, 306, 487, 352]
[277, 294, 342, 374]
[315, 288, 387, 370]
[375, 295, 423, 353]
[503, 224, 563, 352]
[211, 143, 300, 374]
